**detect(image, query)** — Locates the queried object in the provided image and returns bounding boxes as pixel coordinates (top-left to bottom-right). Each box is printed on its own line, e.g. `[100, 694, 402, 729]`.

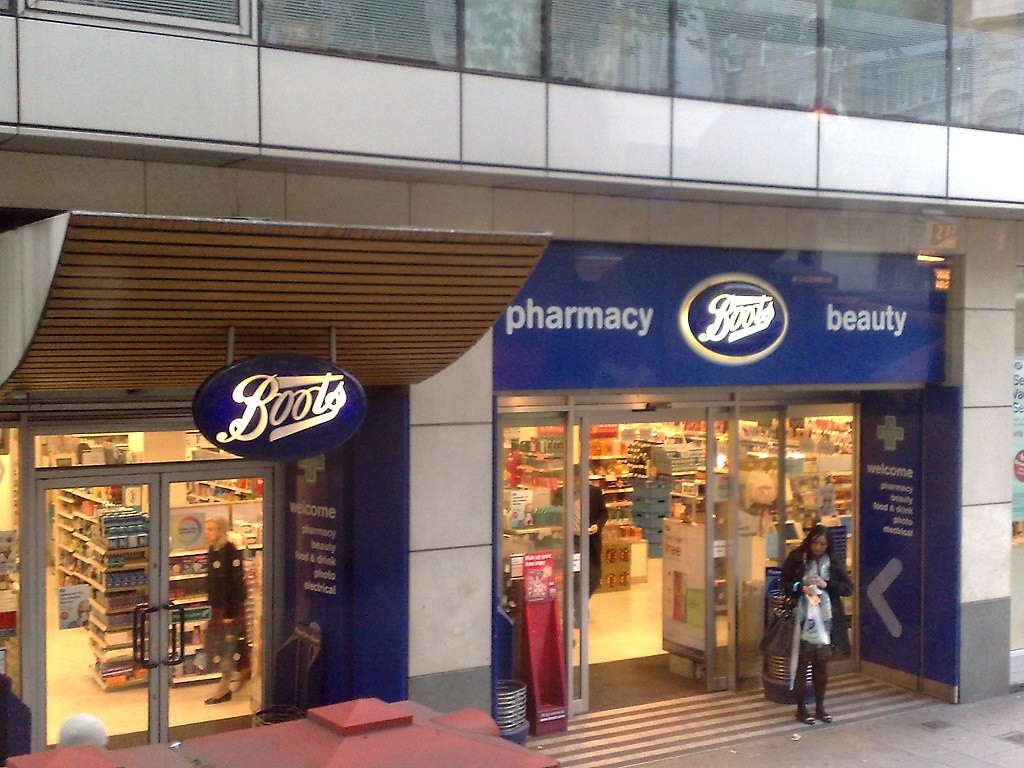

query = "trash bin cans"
[762, 566, 815, 704]
[494, 598, 530, 745]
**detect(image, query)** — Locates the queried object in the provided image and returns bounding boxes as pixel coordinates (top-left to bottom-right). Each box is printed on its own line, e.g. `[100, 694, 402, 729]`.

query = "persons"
[204, 517, 252, 704]
[518, 504, 535, 528]
[781, 526, 853, 725]
[553, 463, 609, 629]
[68, 600, 89, 629]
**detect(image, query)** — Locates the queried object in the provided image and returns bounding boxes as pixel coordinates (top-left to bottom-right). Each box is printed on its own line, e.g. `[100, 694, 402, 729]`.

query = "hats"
[60, 713, 107, 747]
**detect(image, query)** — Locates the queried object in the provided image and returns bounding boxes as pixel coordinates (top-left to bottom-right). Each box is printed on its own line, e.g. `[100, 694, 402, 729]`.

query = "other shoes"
[795, 710, 815, 725]
[815, 708, 832, 722]
[573, 614, 582, 628]
[204, 691, 231, 704]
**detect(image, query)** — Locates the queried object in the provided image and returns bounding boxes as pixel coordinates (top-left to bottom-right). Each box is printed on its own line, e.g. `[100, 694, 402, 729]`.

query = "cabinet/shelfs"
[53, 413, 857, 696]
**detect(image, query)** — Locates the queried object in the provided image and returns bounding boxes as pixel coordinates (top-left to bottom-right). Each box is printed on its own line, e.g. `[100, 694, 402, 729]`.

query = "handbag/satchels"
[801, 596, 832, 645]
[760, 594, 796, 655]
